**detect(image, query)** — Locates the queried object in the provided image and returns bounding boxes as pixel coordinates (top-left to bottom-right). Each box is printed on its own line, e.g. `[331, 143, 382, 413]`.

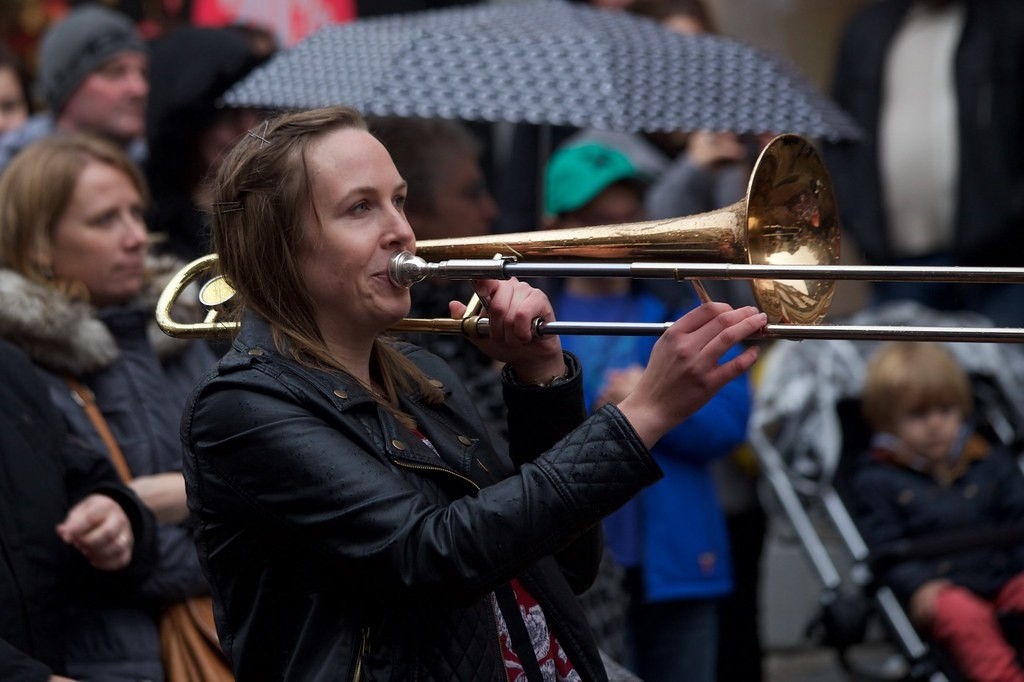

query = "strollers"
[749, 301, 1024, 682]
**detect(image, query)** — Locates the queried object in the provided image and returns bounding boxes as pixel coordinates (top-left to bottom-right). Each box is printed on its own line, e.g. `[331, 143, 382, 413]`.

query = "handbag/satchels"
[156, 595, 235, 682]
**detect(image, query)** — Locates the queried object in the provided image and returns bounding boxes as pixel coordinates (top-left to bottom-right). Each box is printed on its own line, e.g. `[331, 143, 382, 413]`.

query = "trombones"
[153, 127, 1024, 349]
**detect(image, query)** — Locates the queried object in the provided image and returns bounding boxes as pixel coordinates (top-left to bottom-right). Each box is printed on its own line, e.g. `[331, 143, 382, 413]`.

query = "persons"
[542, 142, 752, 682]
[179, 105, 768, 682]
[845, 340, 1024, 682]
[0, 133, 216, 682]
[0, 5, 148, 175]
[827, 0, 1024, 328]
[143, 27, 261, 262]
[368, 115, 506, 431]
[579, 0, 752, 309]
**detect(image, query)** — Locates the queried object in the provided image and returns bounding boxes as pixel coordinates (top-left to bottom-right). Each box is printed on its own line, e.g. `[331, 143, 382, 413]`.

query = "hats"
[38, 9, 148, 120]
[544, 141, 647, 215]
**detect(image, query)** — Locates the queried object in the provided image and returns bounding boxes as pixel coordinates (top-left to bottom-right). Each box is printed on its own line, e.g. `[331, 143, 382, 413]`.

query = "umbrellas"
[225, 0, 870, 142]
